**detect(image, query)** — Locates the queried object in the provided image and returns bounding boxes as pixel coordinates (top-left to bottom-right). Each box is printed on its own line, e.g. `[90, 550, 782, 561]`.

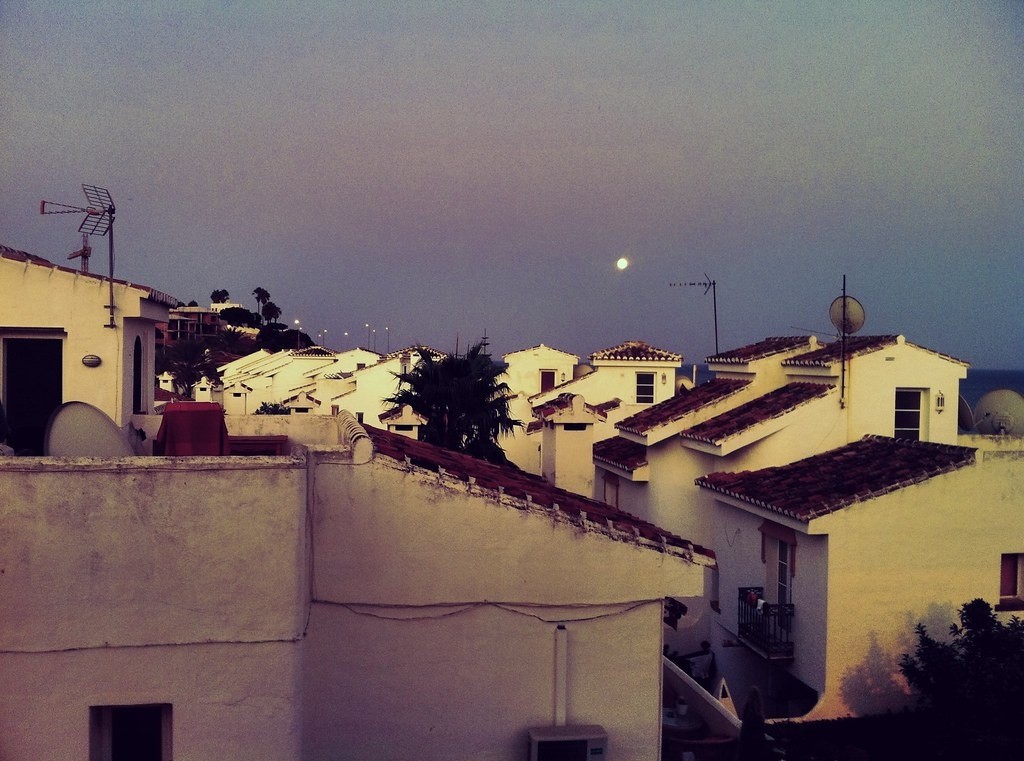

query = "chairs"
[669, 735, 736, 761]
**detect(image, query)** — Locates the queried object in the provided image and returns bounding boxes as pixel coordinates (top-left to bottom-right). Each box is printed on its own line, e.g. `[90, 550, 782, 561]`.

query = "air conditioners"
[528, 724, 608, 760]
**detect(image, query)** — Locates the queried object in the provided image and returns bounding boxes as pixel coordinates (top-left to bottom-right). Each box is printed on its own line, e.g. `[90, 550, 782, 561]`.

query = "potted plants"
[676, 696, 688, 715]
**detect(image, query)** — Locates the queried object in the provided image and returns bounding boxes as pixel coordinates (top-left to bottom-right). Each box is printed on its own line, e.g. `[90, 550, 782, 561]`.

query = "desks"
[662, 700, 703, 734]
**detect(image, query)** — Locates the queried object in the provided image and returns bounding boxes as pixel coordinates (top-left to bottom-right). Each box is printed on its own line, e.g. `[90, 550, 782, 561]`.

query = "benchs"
[229, 435, 289, 456]
